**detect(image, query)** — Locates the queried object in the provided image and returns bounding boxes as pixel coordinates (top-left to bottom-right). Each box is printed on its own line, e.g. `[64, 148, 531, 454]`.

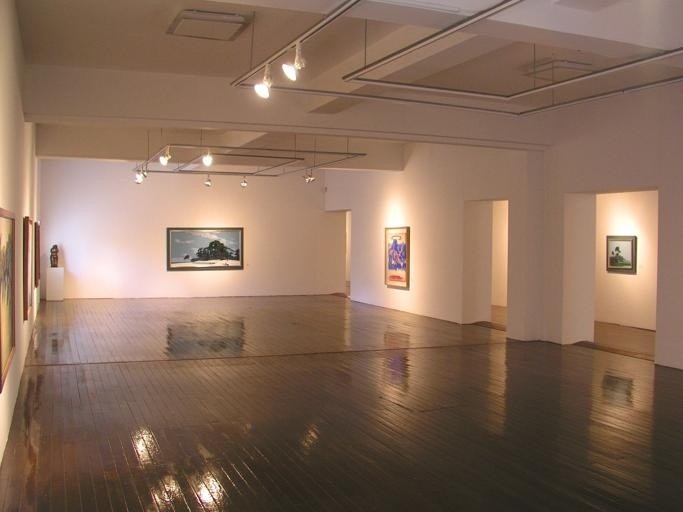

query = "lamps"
[254, 40, 306, 101]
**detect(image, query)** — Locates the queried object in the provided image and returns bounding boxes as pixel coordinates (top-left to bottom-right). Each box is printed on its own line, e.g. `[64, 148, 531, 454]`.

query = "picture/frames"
[385, 228, 410, 288]
[0, 205, 41, 393]
[606, 235, 636, 274]
[166, 226, 244, 271]
